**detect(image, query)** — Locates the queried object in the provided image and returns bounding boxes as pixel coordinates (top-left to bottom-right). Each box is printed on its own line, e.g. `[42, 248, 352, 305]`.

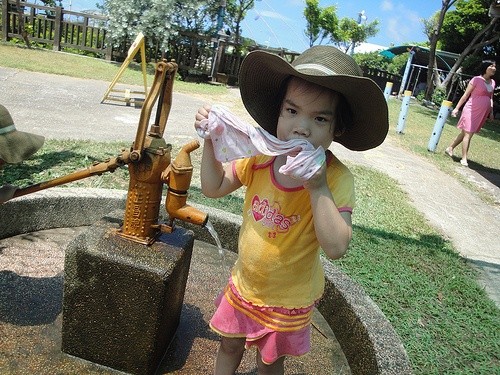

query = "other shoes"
[446, 147, 453, 156]
[460, 159, 469, 166]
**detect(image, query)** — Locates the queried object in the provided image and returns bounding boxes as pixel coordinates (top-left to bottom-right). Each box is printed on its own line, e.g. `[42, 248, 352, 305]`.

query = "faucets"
[165, 191, 208, 228]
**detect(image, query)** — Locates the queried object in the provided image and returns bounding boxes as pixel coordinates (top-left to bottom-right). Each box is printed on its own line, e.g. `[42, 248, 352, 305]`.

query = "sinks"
[0, 196, 377, 375]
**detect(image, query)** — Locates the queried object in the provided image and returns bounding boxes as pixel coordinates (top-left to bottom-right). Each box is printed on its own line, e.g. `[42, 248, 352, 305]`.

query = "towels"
[196, 105, 326, 181]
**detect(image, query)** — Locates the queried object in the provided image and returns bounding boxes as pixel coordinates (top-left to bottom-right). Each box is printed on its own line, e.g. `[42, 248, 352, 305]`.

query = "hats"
[239, 46, 389, 152]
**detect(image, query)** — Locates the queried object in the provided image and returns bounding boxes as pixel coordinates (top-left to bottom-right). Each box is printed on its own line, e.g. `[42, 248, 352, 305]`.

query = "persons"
[0, 104, 46, 205]
[446, 59, 500, 166]
[194, 47, 389, 375]
